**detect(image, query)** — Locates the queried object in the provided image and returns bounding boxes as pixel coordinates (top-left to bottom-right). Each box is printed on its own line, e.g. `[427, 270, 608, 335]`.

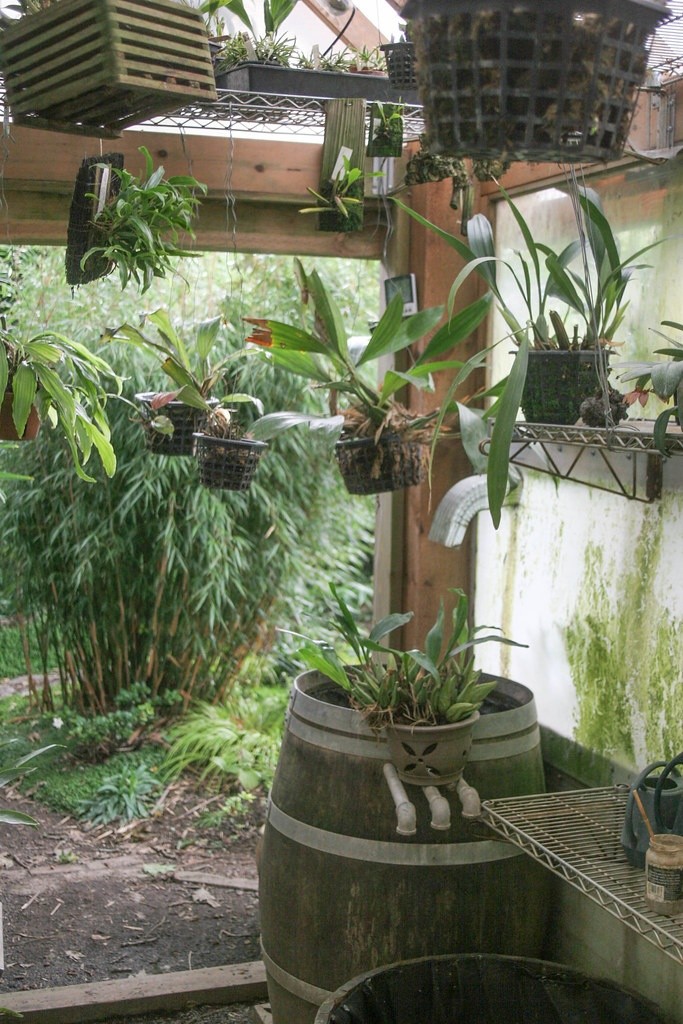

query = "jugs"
[622, 750, 683, 867]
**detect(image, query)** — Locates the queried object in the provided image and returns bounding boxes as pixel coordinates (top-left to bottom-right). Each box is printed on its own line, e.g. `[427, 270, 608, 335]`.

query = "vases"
[0, 0, 219, 141]
[380, 42, 419, 90]
[400, 0, 672, 162]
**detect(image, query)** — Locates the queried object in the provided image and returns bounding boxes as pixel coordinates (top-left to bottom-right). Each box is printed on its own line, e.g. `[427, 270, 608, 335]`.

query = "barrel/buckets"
[258, 663, 550, 1024]
[312, 946, 681, 1024]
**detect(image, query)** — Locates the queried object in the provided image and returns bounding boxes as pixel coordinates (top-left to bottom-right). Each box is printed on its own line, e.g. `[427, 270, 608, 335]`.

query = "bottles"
[645, 834, 683, 916]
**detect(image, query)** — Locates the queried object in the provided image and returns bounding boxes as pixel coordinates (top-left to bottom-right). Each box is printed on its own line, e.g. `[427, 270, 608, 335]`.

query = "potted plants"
[0, 0, 683, 537]
[275, 581, 530, 787]
